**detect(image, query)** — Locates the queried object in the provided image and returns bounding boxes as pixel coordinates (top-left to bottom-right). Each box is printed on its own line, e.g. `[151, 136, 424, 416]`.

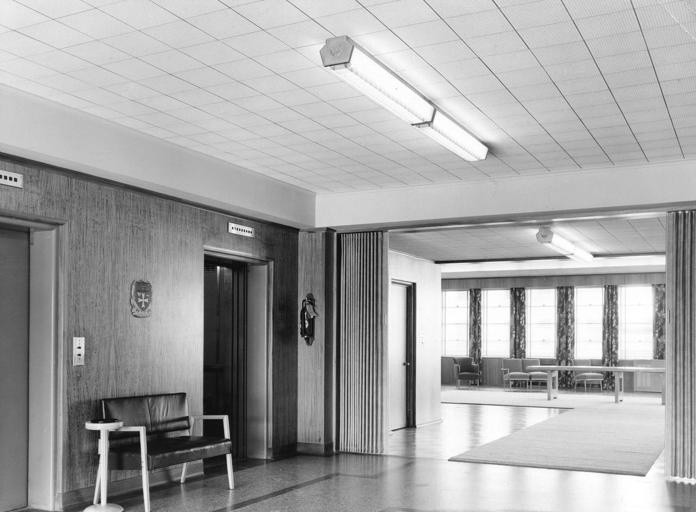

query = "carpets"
[441, 390, 665, 476]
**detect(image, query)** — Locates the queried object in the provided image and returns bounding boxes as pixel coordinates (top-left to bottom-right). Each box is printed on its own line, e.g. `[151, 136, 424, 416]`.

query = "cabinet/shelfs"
[633, 360, 666, 392]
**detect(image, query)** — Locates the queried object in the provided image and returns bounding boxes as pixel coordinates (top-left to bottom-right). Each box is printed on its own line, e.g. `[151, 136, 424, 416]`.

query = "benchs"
[93, 393, 235, 512]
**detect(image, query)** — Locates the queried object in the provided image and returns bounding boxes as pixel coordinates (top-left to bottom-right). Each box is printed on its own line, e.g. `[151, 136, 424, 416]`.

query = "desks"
[527, 366, 666, 405]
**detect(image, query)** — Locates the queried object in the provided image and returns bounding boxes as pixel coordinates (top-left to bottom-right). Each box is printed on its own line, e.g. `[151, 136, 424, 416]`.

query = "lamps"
[536, 229, 594, 265]
[319, 35, 489, 162]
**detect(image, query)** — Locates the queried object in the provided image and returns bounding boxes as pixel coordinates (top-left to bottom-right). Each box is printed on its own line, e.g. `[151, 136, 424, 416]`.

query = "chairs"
[453, 357, 480, 390]
[571, 359, 604, 392]
[522, 359, 552, 392]
[501, 359, 530, 392]
